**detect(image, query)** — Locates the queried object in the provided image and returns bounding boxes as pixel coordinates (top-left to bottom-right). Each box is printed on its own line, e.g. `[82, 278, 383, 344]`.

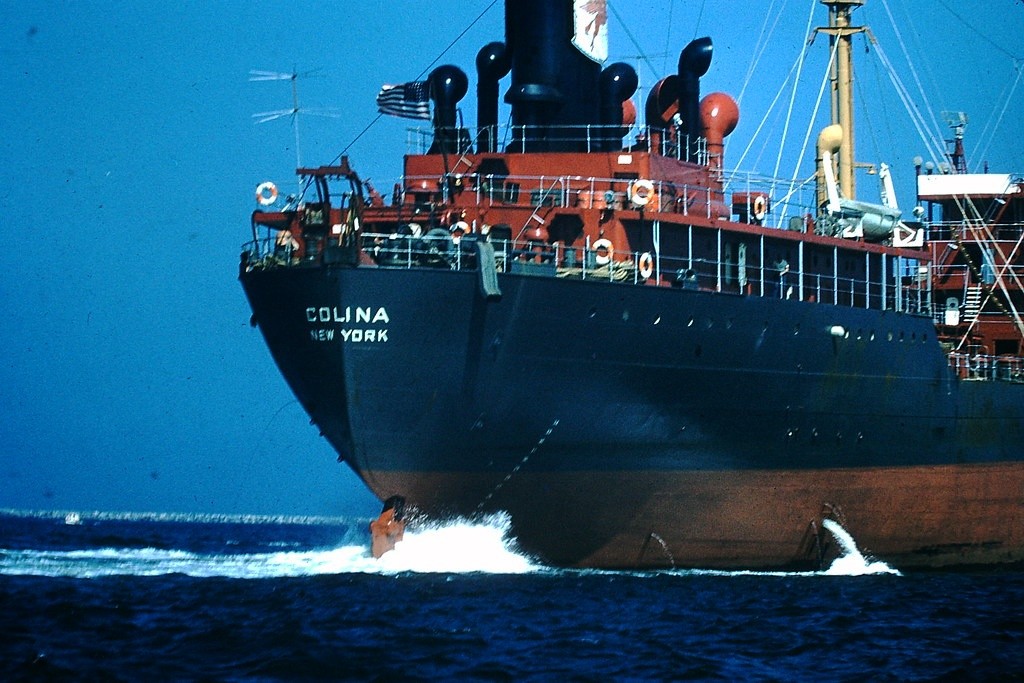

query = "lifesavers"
[591, 237, 614, 264]
[255, 180, 279, 204]
[637, 251, 654, 280]
[449, 220, 474, 242]
[753, 195, 769, 221]
[628, 178, 654, 207]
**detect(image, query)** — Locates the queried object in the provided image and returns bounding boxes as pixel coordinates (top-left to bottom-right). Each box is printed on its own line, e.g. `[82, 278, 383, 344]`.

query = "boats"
[237, 0, 1024, 573]
